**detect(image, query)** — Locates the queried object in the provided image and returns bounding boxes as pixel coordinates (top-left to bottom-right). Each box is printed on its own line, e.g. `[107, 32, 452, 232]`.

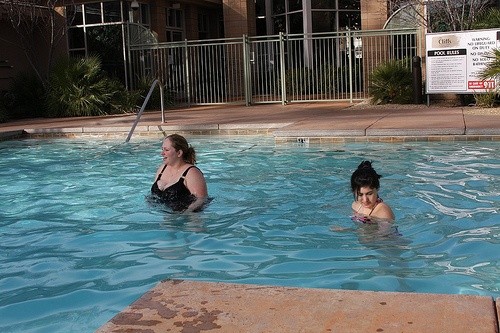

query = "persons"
[351, 160, 397, 221]
[151, 134, 209, 213]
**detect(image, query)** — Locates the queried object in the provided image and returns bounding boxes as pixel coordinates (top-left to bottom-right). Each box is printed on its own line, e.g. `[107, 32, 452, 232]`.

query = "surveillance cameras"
[131, 0, 140, 11]
